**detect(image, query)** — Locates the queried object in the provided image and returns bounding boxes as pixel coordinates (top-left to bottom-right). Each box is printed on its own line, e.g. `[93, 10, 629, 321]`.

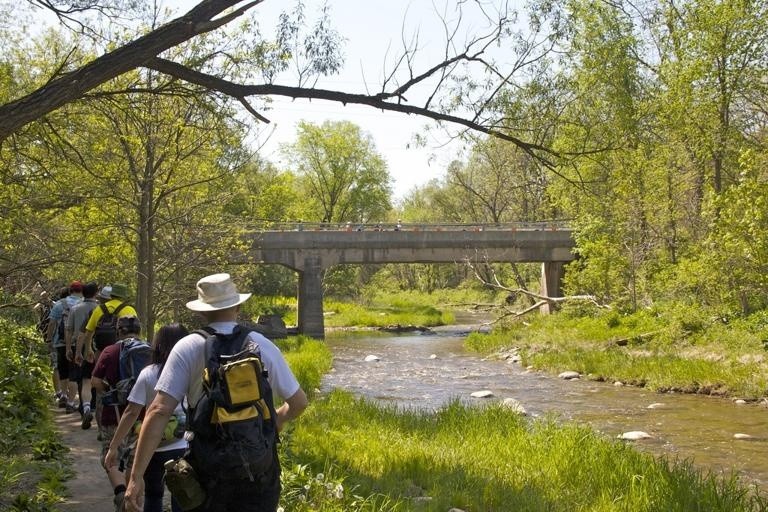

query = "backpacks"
[190, 324, 279, 484]
[115, 339, 157, 404]
[95, 302, 127, 350]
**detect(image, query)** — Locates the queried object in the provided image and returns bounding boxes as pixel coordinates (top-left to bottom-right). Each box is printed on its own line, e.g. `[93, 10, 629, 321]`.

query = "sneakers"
[81, 412, 94, 430]
[58, 398, 67, 408]
[66, 405, 76, 414]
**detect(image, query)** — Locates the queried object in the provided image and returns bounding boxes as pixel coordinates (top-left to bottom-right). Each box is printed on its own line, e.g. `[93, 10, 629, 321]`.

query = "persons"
[36, 278, 139, 442]
[123, 272, 309, 511]
[103, 322, 196, 512]
[88, 313, 156, 512]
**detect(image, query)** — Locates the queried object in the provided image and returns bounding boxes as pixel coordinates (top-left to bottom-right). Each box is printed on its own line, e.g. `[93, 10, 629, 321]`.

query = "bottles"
[163, 457, 207, 511]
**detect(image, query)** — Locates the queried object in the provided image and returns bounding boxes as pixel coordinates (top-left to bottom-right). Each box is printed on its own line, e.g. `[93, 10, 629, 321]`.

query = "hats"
[184, 272, 253, 313]
[110, 283, 128, 301]
[99, 286, 113, 299]
[83, 281, 96, 297]
[118, 316, 141, 331]
[70, 280, 81, 289]
